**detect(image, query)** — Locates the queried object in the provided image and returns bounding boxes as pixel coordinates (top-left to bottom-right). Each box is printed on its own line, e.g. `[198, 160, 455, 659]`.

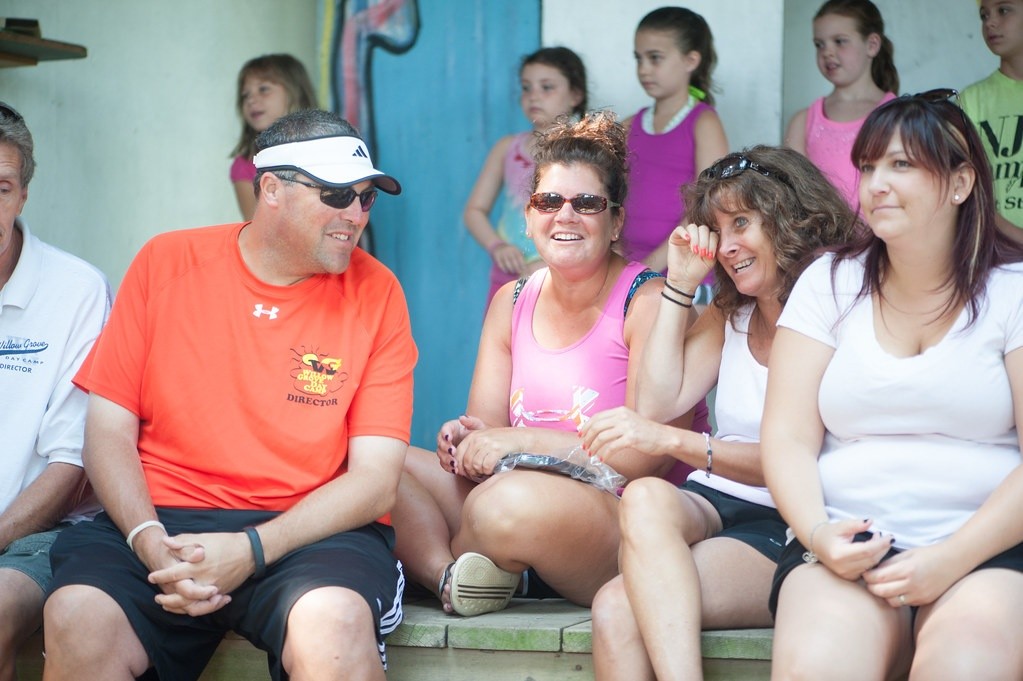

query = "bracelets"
[126, 521, 167, 551]
[240, 526, 266, 579]
[704, 432, 714, 477]
[664, 281, 695, 299]
[660, 292, 693, 309]
[802, 523, 828, 566]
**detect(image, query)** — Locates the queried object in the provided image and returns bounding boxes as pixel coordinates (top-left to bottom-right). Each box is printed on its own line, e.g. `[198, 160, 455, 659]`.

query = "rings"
[900, 595, 907, 607]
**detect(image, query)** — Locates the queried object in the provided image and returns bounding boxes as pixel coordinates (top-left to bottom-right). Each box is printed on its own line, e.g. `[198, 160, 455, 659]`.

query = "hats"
[253, 135, 402, 195]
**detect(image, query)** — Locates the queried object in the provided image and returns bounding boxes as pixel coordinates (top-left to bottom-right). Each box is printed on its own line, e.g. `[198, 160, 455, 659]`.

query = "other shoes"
[437, 552, 522, 618]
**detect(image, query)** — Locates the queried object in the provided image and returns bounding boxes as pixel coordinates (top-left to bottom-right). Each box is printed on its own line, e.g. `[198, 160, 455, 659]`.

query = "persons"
[390, 109, 712, 616]
[948, 0, 1023, 244]
[42, 109, 422, 681]
[787, 0, 902, 239]
[464, 47, 588, 322]
[615, 7, 730, 314]
[0, 102, 113, 681]
[761, 88, 1023, 681]
[230, 53, 321, 222]
[588, 147, 857, 681]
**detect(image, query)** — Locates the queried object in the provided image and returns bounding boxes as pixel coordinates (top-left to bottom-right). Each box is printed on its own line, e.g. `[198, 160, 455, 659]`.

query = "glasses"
[278, 178, 380, 214]
[697, 156, 806, 217]
[529, 191, 622, 215]
[897, 88, 974, 164]
